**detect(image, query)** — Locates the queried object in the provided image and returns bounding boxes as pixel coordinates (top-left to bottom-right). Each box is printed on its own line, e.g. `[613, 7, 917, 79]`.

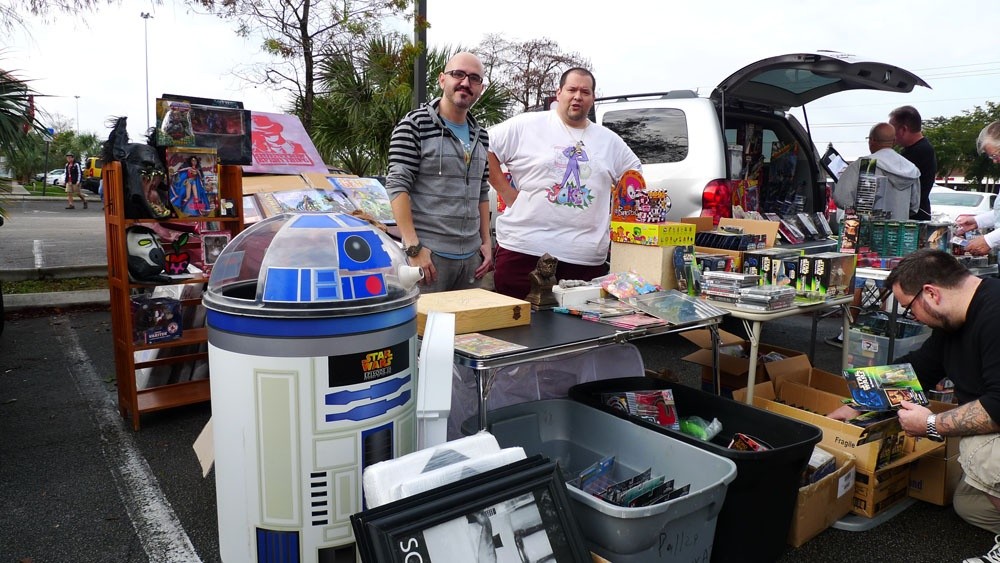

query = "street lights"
[73, 96, 82, 136]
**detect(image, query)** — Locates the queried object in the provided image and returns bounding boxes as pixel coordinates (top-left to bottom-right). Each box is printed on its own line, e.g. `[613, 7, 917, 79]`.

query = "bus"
[84, 156, 103, 178]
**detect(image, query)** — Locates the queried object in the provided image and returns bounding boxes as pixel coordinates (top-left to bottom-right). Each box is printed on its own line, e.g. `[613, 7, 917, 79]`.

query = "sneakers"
[962, 533, 1000, 563]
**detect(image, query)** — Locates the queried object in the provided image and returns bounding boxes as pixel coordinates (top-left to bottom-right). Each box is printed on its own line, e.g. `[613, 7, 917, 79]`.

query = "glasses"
[444, 70, 483, 85]
[901, 281, 936, 321]
[988, 151, 1000, 160]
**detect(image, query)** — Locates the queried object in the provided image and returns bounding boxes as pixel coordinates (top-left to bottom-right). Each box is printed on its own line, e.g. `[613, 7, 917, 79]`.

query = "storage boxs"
[419, 218, 959, 563]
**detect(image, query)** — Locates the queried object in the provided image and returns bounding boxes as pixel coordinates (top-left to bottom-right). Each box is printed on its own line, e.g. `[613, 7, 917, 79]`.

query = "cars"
[34, 168, 65, 186]
[922, 183, 998, 230]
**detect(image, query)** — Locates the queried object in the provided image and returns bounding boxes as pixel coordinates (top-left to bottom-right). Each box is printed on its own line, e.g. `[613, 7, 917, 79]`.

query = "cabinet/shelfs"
[103, 161, 244, 430]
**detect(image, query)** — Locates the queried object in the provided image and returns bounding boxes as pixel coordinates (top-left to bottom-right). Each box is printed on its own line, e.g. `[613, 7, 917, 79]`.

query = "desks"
[700, 275, 855, 405]
[417, 292, 723, 438]
[856, 263, 999, 364]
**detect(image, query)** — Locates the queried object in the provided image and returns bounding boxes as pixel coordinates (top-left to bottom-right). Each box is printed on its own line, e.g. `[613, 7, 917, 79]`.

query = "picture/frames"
[349, 451, 592, 563]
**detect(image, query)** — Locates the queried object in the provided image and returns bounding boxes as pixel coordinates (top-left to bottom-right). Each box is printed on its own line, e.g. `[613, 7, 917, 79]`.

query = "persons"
[833, 122, 921, 220]
[828, 248, 1000, 563]
[385, 52, 492, 294]
[955, 121, 1000, 256]
[178, 156, 202, 210]
[890, 106, 936, 221]
[486, 67, 642, 301]
[64, 152, 88, 209]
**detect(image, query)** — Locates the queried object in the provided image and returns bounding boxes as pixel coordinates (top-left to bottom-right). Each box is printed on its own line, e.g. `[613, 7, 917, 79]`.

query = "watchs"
[405, 242, 423, 257]
[927, 414, 945, 442]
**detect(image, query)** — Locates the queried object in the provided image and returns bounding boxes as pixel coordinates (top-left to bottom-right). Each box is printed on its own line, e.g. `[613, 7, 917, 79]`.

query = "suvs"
[526, 49, 934, 257]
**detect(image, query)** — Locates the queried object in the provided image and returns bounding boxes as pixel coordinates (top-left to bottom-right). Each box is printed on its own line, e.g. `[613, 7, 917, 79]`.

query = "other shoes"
[823, 327, 843, 348]
[65, 204, 75, 209]
[83, 201, 88, 209]
